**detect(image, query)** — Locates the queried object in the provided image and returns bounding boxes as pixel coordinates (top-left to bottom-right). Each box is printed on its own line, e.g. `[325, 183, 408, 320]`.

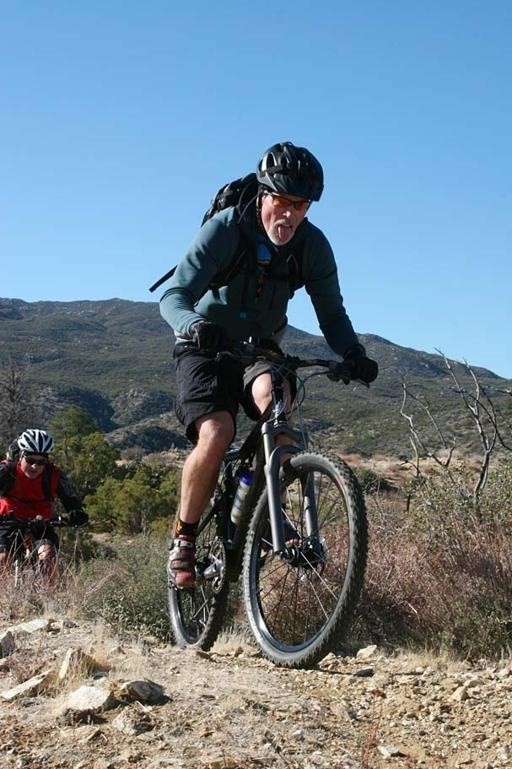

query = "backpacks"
[148, 173, 307, 301]
[1, 438, 18, 494]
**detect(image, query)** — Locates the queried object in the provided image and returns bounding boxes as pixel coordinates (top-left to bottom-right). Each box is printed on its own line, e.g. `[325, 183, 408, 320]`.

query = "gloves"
[342, 343, 378, 382]
[67, 509, 89, 527]
[190, 319, 226, 354]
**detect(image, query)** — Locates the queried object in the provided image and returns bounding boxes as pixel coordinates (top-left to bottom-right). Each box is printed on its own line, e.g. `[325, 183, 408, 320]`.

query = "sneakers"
[167, 505, 200, 592]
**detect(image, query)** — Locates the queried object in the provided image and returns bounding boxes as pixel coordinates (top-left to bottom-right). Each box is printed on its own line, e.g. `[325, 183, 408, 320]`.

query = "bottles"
[230, 475, 252, 525]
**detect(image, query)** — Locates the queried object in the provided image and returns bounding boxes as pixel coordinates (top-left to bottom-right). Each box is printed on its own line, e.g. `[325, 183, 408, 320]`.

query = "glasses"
[23, 456, 47, 467]
[268, 191, 312, 211]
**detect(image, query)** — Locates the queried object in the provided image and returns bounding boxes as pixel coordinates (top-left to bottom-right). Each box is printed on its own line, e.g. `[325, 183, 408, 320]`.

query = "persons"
[154, 140, 379, 587]
[0, 428, 87, 592]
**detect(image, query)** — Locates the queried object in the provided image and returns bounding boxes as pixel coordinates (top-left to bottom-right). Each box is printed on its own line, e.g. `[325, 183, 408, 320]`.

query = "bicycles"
[0, 511, 87, 593]
[165, 331, 375, 671]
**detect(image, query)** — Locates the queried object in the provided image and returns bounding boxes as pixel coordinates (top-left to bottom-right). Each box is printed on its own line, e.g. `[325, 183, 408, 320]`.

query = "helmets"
[16, 428, 55, 454]
[256, 142, 324, 201]
